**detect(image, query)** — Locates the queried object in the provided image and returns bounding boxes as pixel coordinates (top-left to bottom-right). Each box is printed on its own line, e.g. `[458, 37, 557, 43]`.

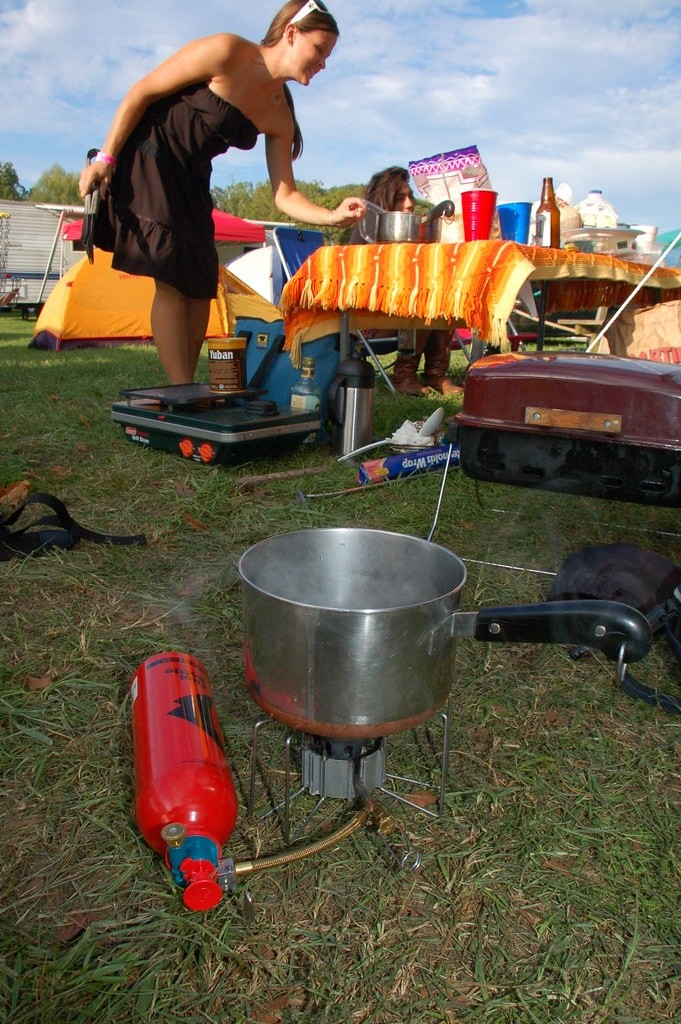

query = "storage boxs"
[354, 442, 461, 486]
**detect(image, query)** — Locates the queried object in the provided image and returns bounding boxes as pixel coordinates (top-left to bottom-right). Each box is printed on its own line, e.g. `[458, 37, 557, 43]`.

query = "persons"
[348, 166, 465, 397]
[77, 0, 366, 385]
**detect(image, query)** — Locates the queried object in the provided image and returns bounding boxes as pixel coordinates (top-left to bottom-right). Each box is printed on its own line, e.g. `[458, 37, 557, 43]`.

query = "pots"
[238, 527, 654, 739]
[375, 200, 454, 243]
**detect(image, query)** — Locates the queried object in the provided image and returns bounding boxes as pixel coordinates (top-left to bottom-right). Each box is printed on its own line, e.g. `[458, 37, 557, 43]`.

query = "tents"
[27, 197, 285, 355]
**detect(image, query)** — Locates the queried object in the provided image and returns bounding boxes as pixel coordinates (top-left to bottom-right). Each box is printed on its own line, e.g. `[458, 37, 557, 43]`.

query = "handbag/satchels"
[545, 542, 681, 616]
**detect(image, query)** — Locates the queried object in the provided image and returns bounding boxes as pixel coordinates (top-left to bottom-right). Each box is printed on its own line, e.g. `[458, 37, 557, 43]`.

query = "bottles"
[130, 651, 239, 909]
[573, 190, 618, 229]
[537, 177, 560, 248]
[290, 356, 323, 412]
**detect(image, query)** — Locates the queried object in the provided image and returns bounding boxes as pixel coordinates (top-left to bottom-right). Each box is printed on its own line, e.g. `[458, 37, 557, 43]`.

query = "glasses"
[283, 0, 331, 38]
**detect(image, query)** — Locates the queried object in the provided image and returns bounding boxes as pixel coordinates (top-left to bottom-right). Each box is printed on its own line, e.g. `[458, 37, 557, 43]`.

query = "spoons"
[336, 407, 445, 461]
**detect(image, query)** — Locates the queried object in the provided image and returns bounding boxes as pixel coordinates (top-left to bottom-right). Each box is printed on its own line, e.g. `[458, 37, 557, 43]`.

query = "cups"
[495, 202, 533, 245]
[630, 225, 659, 255]
[461, 189, 498, 242]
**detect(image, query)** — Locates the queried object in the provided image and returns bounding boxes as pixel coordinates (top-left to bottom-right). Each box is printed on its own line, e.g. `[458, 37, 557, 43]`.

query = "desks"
[277, 241, 680, 369]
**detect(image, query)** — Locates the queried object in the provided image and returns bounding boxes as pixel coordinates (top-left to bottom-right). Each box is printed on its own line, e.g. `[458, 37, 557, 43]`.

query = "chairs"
[272, 224, 526, 393]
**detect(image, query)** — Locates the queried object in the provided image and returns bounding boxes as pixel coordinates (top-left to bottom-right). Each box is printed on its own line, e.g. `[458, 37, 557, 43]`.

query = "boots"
[388, 330, 432, 397]
[425, 330, 465, 396]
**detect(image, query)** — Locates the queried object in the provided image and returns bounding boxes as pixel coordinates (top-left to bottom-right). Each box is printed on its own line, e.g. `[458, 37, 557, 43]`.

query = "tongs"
[81, 149, 100, 265]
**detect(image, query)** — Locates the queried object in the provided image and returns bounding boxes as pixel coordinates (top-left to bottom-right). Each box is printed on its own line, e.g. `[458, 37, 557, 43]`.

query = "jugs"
[327, 359, 375, 457]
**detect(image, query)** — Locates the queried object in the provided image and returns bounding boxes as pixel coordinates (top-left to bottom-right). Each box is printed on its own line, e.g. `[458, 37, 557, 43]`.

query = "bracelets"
[96, 150, 116, 168]
[330, 209, 337, 227]
